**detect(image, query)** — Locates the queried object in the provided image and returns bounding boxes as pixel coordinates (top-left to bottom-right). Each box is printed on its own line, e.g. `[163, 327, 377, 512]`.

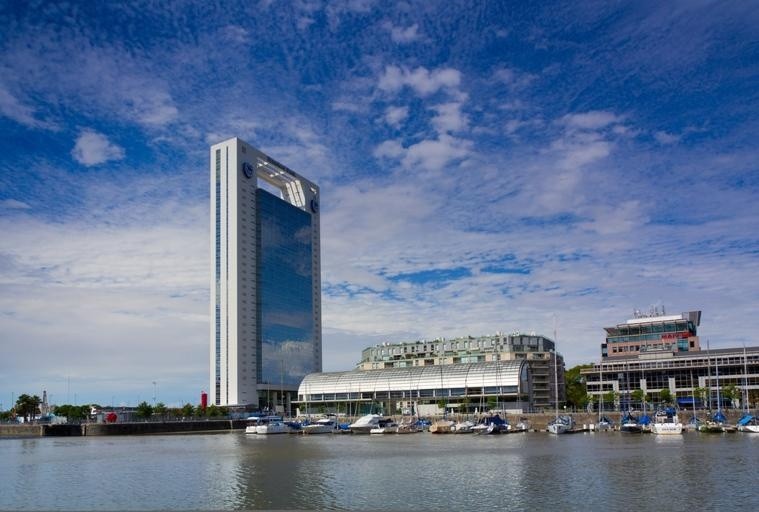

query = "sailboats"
[546, 315, 567, 434]
[595, 339, 759, 435]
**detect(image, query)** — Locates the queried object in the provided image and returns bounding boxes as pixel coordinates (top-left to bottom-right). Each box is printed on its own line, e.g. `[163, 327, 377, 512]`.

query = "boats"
[244, 414, 531, 435]
